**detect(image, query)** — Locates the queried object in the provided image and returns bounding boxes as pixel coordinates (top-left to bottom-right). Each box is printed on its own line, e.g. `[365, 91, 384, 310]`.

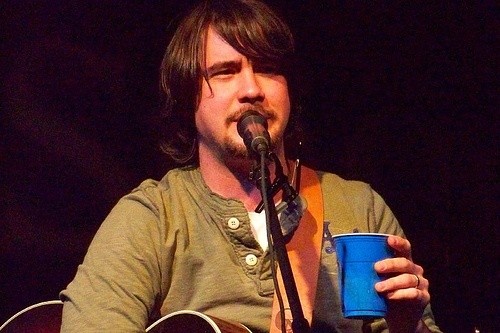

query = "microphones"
[237, 109, 271, 154]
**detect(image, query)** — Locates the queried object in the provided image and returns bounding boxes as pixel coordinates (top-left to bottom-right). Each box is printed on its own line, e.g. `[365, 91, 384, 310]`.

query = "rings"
[410, 273, 421, 290]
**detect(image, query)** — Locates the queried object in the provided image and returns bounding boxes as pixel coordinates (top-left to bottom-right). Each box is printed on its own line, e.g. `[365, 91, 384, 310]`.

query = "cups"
[331, 232, 396, 320]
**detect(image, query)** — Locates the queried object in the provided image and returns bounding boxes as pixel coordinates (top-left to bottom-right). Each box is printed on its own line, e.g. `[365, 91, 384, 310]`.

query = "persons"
[59, 1, 447, 333]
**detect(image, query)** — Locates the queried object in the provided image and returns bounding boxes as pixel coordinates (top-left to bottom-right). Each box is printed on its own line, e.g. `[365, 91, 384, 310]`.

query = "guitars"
[0, 301, 251, 333]
